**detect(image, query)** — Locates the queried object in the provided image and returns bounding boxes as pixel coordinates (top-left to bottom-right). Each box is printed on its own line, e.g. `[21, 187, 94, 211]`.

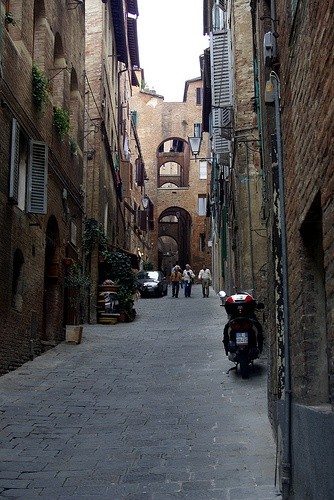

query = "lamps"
[134, 193, 148, 211]
[186, 136, 215, 161]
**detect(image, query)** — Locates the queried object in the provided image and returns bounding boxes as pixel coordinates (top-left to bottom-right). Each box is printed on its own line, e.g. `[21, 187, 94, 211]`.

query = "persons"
[182, 263, 196, 297]
[198, 264, 212, 298]
[170, 265, 182, 298]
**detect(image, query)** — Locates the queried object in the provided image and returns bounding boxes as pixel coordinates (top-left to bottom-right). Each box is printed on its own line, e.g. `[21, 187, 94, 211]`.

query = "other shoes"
[172, 294, 175, 297]
[203, 296, 205, 298]
[188, 295, 191, 297]
[175, 295, 178, 298]
[185, 295, 187, 297]
[206, 295, 208, 297]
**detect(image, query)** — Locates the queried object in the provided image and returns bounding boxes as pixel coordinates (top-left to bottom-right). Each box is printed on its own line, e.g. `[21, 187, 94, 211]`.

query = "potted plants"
[64, 262, 92, 343]
[118, 292, 134, 321]
[98, 249, 106, 263]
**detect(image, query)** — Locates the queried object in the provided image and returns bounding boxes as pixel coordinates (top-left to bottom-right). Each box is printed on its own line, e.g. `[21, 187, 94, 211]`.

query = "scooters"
[218, 288, 267, 377]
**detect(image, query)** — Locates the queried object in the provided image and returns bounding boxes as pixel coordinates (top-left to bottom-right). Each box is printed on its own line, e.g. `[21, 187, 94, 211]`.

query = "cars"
[135, 270, 169, 298]
